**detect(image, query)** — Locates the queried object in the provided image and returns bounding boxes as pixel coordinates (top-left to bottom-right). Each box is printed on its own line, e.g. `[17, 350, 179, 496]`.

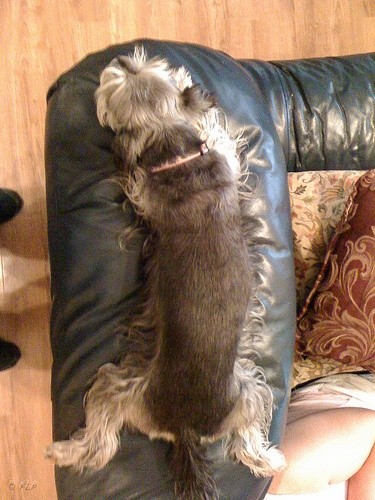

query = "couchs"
[44, 47, 375, 500]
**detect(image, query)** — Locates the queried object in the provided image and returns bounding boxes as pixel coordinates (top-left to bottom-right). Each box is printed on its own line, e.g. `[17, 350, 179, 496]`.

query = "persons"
[0, 187, 23, 373]
[263, 406, 375, 499]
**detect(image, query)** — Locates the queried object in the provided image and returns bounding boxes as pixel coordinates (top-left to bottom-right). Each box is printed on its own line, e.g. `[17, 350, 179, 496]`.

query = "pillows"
[293, 168, 375, 375]
[286, 171, 368, 392]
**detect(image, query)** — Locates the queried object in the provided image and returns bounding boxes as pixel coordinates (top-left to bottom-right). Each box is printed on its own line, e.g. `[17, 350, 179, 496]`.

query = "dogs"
[43, 45, 287, 500]
